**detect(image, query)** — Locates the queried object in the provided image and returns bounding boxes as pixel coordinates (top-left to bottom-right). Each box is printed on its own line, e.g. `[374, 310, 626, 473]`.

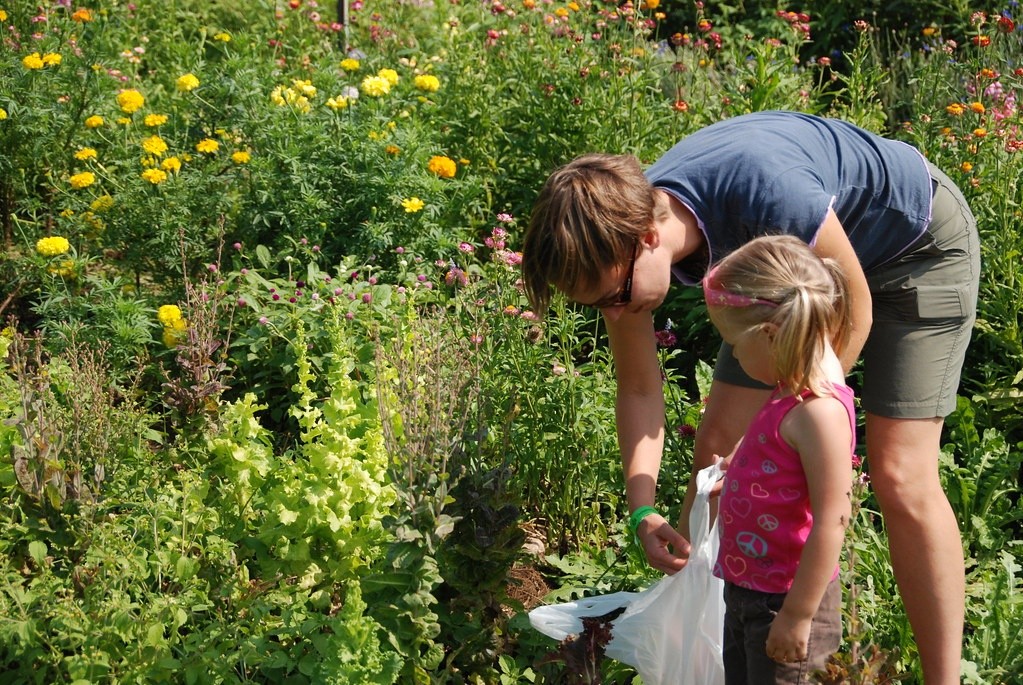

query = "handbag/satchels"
[530, 455, 724, 685]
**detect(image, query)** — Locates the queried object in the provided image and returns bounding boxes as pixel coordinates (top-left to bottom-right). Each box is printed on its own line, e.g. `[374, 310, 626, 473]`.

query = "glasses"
[582, 236, 638, 312]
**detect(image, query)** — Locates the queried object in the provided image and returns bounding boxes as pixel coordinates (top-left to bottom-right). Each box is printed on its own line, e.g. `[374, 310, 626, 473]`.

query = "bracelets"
[630, 504, 659, 542]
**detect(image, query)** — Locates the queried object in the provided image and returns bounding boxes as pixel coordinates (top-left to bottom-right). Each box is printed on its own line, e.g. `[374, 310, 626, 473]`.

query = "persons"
[703, 233, 857, 685]
[520, 109, 981, 685]
